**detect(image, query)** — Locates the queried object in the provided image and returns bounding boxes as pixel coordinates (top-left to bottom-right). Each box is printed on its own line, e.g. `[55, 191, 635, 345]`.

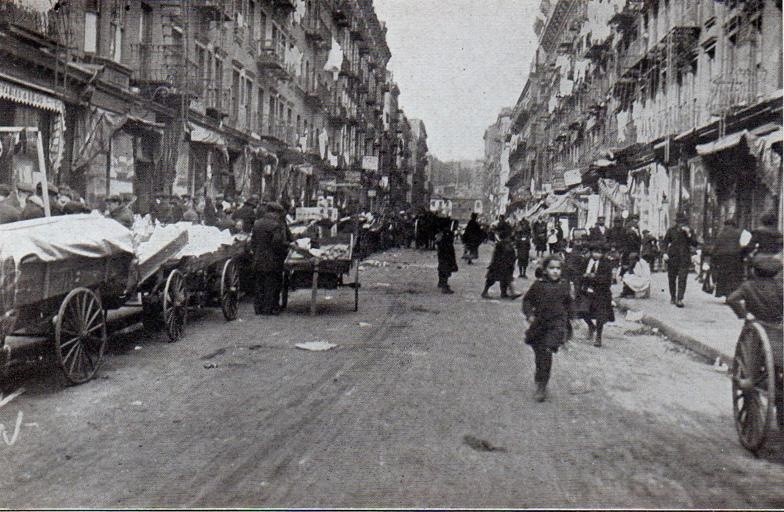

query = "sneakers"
[481, 290, 522, 300]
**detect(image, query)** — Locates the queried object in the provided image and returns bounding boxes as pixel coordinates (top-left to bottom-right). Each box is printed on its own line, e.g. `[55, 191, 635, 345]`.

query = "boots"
[534, 382, 544, 401]
[671, 296, 683, 307]
[437, 280, 454, 294]
[589, 323, 602, 347]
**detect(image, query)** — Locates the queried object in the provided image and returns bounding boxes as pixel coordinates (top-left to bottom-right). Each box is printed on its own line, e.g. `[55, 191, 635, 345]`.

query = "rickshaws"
[725, 294, 783, 452]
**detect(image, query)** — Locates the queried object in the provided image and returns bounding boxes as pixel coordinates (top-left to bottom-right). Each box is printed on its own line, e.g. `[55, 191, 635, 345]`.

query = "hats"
[37, 180, 59, 195]
[673, 213, 686, 222]
[105, 195, 122, 201]
[754, 254, 783, 272]
[17, 182, 35, 194]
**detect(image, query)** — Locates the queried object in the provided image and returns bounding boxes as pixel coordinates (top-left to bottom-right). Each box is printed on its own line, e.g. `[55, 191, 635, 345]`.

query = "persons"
[353, 206, 658, 300]
[522, 256, 572, 401]
[574, 241, 615, 346]
[2, 184, 289, 316]
[661, 211, 784, 326]
